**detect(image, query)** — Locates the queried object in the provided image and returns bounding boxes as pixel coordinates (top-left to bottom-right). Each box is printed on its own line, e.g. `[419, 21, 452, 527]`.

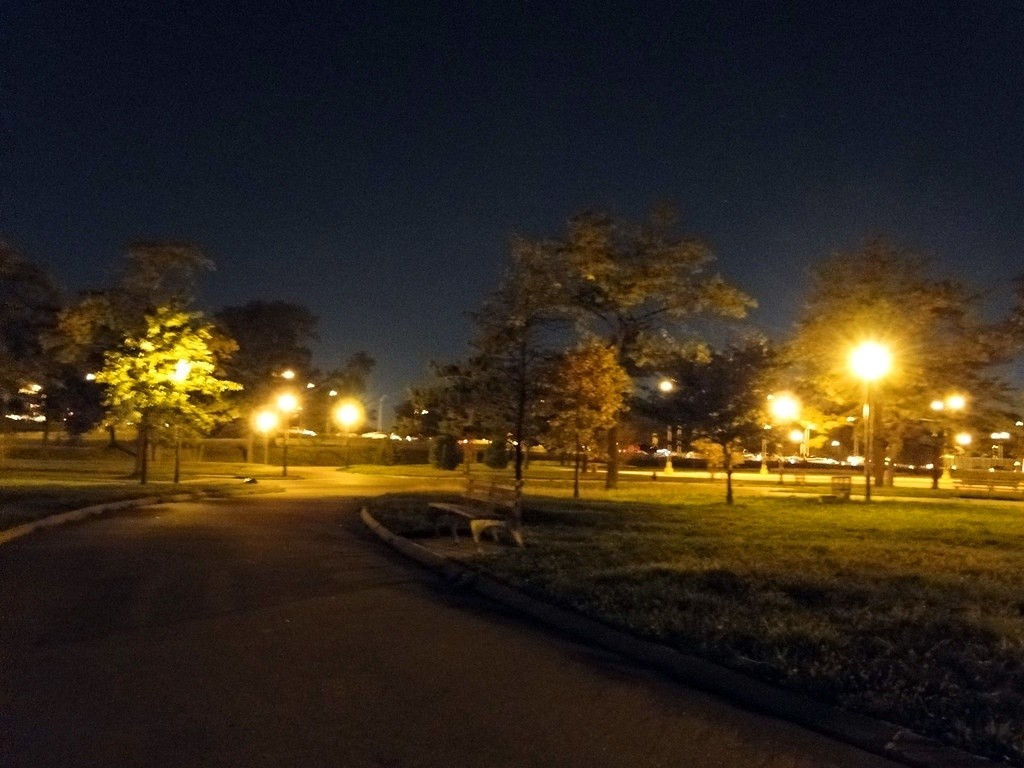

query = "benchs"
[949, 469, 1024, 492]
[428, 473, 527, 556]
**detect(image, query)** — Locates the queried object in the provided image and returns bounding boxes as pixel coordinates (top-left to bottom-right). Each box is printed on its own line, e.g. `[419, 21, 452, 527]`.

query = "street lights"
[253, 411, 280, 467]
[849, 339, 888, 503]
[772, 392, 797, 483]
[170, 358, 190, 485]
[335, 402, 360, 470]
[276, 390, 298, 474]
